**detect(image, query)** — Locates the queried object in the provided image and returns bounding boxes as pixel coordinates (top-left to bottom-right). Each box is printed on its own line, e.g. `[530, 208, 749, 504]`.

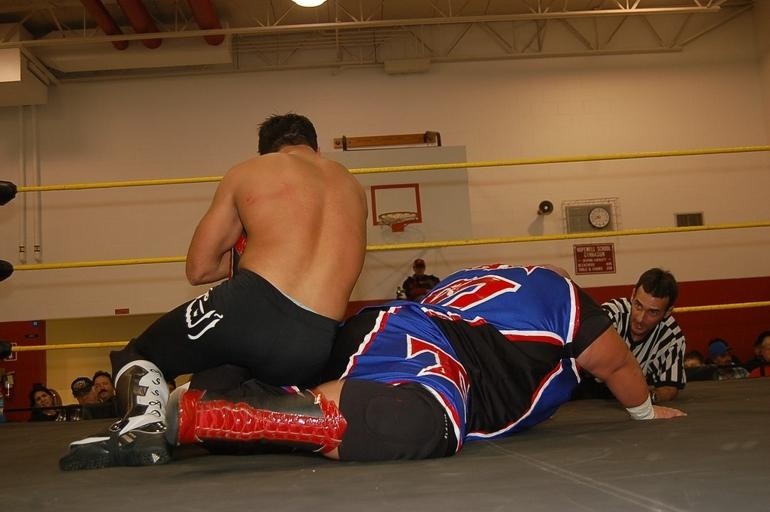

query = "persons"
[163, 262, 692, 466]
[700, 340, 750, 381]
[26, 384, 61, 421]
[403, 257, 442, 297]
[71, 376, 98, 408]
[89, 370, 115, 398]
[750, 333, 769, 378]
[684, 349, 706, 366]
[594, 265, 687, 402]
[743, 347, 762, 371]
[52, 110, 374, 475]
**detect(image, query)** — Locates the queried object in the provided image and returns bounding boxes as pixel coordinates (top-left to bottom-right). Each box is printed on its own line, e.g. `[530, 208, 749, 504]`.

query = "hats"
[71, 377, 93, 395]
[708, 341, 729, 357]
[414, 259, 425, 268]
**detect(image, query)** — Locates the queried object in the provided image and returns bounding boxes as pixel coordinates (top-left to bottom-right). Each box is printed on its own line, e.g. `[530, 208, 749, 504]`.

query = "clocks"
[588, 206, 610, 229]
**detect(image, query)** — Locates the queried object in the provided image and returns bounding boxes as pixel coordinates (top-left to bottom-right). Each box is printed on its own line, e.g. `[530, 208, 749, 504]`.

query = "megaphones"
[538, 201, 554, 216]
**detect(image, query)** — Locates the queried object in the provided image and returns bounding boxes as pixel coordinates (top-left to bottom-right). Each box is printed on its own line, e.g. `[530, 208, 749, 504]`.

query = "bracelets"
[650, 390, 658, 404]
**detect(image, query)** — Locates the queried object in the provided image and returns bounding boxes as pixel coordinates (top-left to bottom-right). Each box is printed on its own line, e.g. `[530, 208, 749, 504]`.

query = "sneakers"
[60, 424, 170, 473]
[168, 381, 202, 448]
